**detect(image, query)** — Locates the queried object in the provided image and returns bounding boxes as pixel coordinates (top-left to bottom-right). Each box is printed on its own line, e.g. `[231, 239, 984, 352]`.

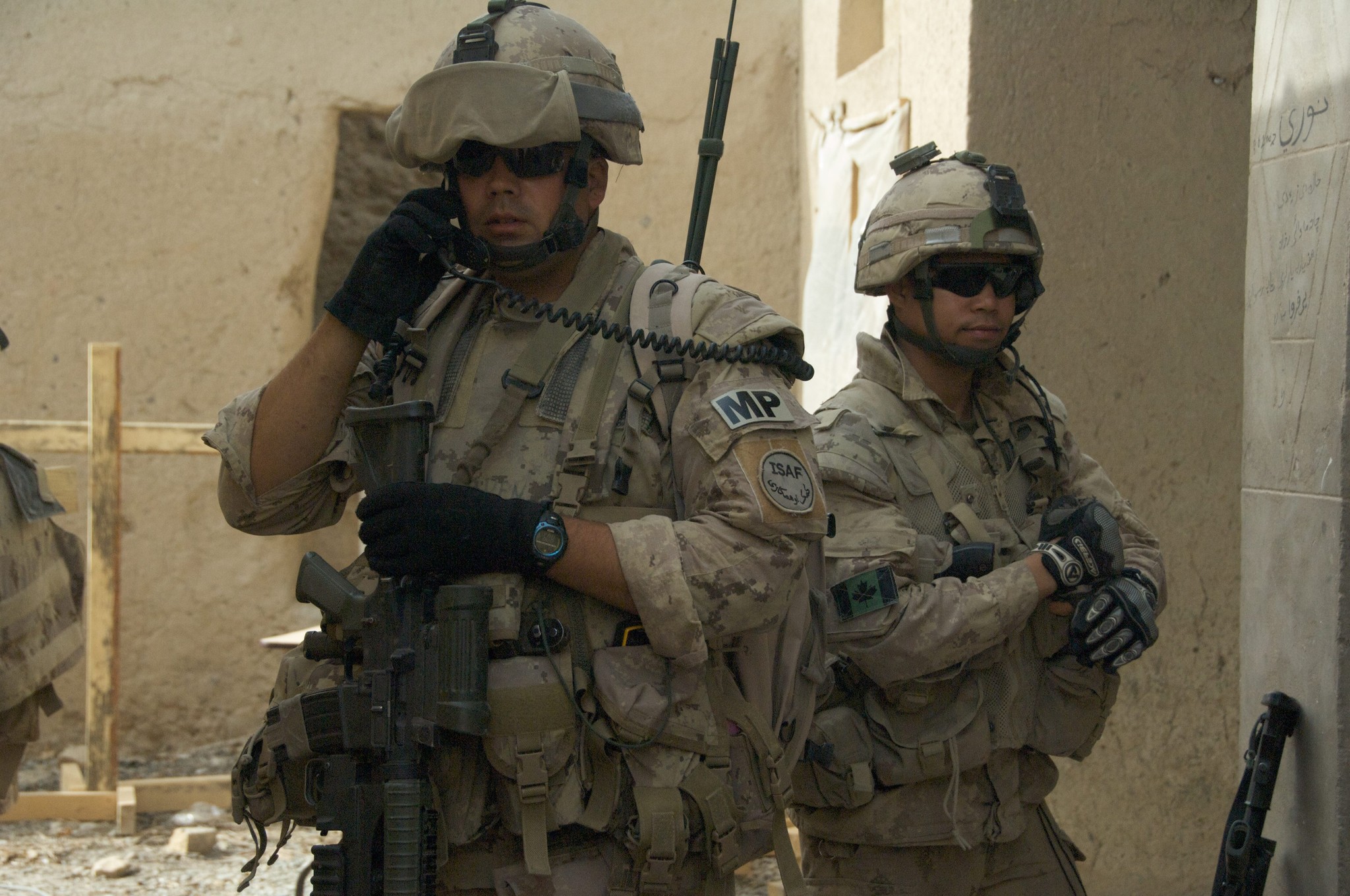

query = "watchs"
[522, 510, 569, 579]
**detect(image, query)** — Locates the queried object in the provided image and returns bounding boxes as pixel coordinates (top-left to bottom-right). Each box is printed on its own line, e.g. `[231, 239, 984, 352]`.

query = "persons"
[201, 0, 827, 896]
[0, 331, 89, 815]
[785, 139, 1165, 896]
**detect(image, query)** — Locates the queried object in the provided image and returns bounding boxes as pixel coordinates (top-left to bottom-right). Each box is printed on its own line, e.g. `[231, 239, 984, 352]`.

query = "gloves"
[1034, 499, 1125, 593]
[323, 186, 469, 340]
[1069, 575, 1159, 671]
[353, 481, 551, 586]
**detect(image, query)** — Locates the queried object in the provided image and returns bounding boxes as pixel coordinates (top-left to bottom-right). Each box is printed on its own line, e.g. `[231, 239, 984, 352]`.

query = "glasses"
[458, 140, 573, 179]
[907, 262, 1024, 298]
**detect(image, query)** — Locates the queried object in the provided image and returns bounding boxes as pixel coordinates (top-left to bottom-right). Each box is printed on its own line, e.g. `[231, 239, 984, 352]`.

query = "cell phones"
[611, 620, 649, 646]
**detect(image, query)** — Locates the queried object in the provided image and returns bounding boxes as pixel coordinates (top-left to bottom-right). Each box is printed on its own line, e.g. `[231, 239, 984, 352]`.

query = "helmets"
[853, 155, 1044, 315]
[381, 2, 646, 167]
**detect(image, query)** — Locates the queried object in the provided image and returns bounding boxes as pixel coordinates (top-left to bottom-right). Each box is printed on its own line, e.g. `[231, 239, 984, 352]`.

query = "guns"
[1210, 691, 1302, 896]
[263, 400, 494, 896]
